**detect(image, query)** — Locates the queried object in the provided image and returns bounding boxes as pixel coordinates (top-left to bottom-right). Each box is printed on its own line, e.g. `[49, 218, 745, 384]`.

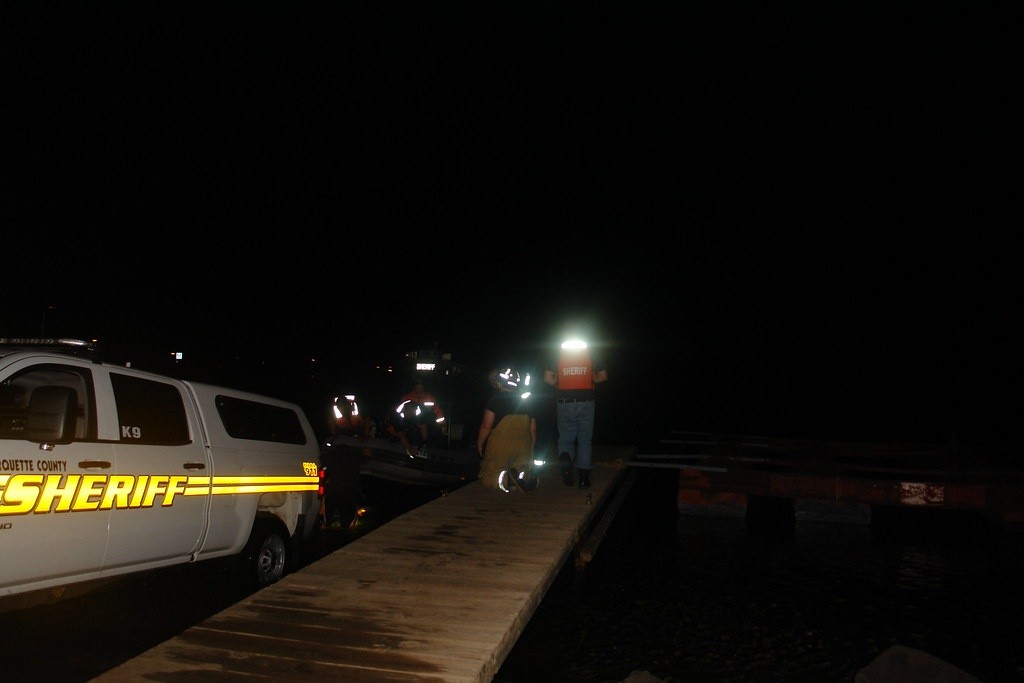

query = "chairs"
[24, 386, 78, 439]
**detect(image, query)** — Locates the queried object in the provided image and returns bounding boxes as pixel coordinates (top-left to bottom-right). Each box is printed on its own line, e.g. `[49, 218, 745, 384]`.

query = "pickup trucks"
[0, 351, 327, 600]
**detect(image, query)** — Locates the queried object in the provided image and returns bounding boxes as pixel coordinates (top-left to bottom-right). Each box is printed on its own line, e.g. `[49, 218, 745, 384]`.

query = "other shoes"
[560, 451, 574, 487]
[415, 447, 431, 459]
[574, 465, 592, 489]
[529, 468, 539, 489]
[508, 468, 529, 494]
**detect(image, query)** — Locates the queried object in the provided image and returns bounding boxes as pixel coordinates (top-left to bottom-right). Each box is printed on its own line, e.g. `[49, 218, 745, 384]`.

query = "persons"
[476, 367, 538, 496]
[325, 396, 372, 439]
[543, 347, 608, 491]
[376, 379, 447, 455]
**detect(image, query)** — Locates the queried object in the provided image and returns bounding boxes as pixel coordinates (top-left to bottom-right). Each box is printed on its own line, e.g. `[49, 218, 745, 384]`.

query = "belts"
[558, 398, 593, 402]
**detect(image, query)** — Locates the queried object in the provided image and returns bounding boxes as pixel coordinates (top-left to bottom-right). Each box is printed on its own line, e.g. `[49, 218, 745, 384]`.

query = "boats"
[594, 428, 995, 519]
[319, 413, 485, 489]
